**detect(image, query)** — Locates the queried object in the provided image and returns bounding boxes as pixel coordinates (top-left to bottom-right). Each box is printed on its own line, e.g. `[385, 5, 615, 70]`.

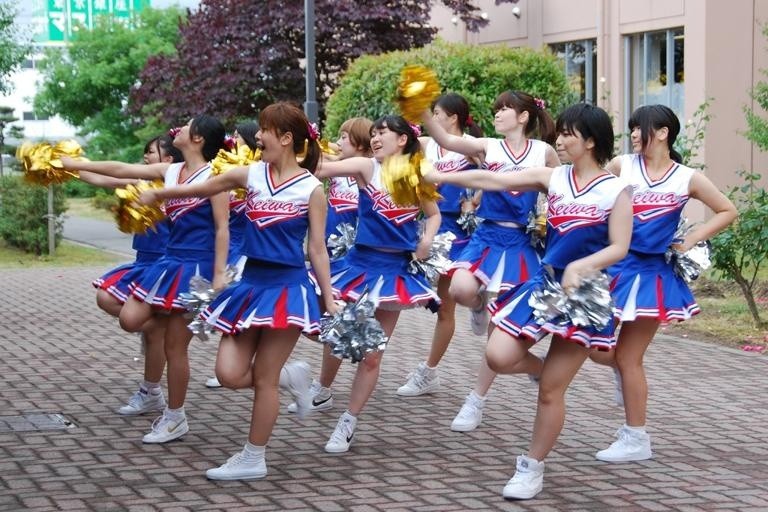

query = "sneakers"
[285, 363, 356, 452]
[205, 377, 220, 388]
[596, 424, 652, 462]
[205, 447, 267, 480]
[118, 383, 189, 444]
[503, 456, 544, 499]
[397, 361, 440, 396]
[451, 394, 482, 432]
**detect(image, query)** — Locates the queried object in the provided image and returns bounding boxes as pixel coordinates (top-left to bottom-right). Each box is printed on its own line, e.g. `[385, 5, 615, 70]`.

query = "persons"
[291, 116, 442, 454]
[53, 100, 345, 480]
[395, 93, 633, 500]
[589, 105, 738, 462]
